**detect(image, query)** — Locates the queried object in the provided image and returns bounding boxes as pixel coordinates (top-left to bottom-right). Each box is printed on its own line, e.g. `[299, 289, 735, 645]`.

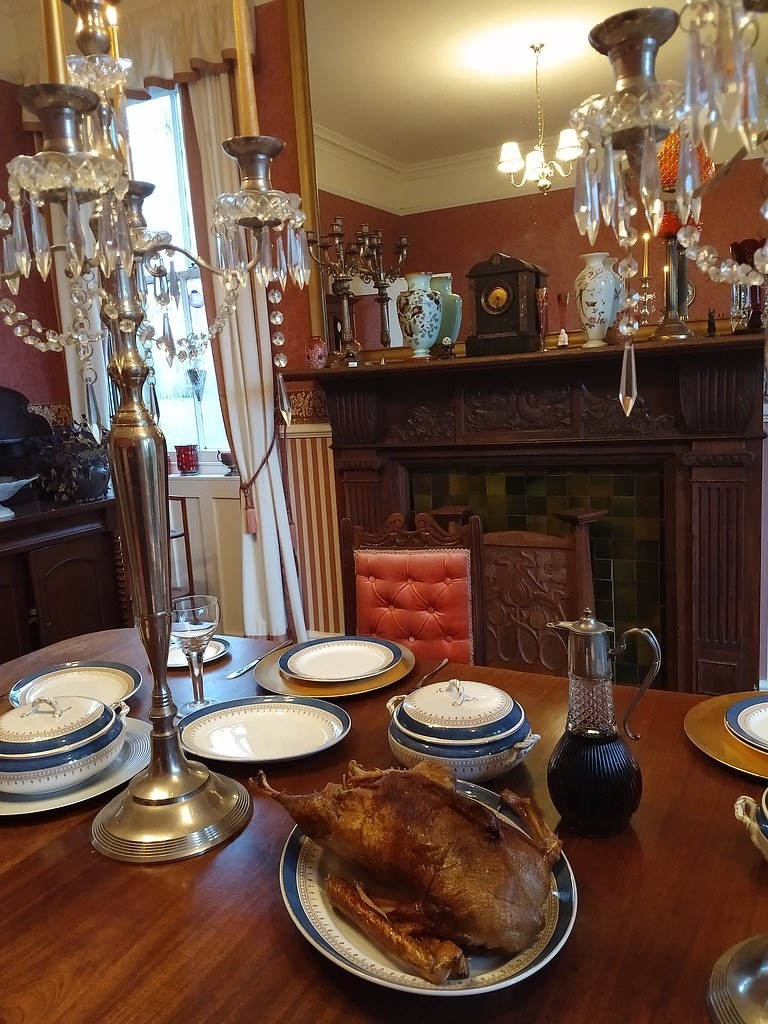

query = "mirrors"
[287, 0, 768, 367]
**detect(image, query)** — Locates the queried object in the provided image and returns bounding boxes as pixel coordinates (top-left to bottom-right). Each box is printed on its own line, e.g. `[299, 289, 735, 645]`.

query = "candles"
[232, 0, 259, 136]
[109, 26, 133, 178]
[39, 1, 71, 85]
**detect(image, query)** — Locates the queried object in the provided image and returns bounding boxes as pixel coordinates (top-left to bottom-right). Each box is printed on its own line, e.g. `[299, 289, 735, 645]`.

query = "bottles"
[430, 275, 462, 343]
[305, 336, 328, 370]
[602, 256, 623, 326]
[574, 252, 614, 348]
[395, 271, 443, 358]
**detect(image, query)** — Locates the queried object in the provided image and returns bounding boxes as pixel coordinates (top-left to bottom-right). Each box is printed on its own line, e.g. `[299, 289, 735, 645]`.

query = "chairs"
[335, 511, 488, 669]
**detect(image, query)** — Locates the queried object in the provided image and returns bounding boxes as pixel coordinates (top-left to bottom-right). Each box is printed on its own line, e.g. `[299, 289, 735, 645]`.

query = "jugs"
[547, 608, 660, 840]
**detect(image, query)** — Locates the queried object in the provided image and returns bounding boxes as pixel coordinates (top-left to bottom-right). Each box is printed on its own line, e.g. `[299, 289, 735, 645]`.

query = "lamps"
[638, 231, 668, 325]
[496, 45, 584, 196]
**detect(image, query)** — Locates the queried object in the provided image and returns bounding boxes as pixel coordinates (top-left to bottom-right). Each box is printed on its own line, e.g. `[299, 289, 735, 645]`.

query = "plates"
[166, 637, 231, 668]
[684, 691, 768, 780]
[0, 717, 152, 816]
[723, 695, 768, 756]
[279, 780, 578, 997]
[8, 660, 142, 706]
[252, 640, 416, 698]
[277, 636, 403, 683]
[176, 695, 352, 764]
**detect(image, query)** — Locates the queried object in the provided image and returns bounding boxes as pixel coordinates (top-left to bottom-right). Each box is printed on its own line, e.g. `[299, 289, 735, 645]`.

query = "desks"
[0, 626, 768, 1024]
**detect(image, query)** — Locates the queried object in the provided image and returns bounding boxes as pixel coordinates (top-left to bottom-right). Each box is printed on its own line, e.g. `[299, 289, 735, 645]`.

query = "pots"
[0, 696, 130, 796]
[386, 676, 540, 780]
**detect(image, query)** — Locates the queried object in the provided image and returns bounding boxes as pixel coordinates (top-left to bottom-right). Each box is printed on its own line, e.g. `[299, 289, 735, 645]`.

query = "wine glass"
[170, 594, 221, 717]
[216, 450, 238, 476]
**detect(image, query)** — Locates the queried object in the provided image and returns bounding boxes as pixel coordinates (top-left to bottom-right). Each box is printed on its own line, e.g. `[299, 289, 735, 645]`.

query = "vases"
[573, 253, 614, 348]
[174, 445, 199, 476]
[305, 335, 328, 370]
[431, 275, 462, 344]
[603, 258, 626, 327]
[396, 271, 443, 359]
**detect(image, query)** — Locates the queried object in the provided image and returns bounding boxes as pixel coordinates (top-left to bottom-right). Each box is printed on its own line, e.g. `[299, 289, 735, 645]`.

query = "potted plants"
[12, 414, 111, 506]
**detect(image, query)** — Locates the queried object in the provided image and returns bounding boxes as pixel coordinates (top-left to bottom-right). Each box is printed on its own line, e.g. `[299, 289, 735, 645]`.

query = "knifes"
[225, 639, 293, 679]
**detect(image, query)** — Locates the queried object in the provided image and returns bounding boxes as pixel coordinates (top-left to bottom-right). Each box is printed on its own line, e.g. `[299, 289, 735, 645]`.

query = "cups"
[730, 237, 768, 333]
[174, 444, 199, 476]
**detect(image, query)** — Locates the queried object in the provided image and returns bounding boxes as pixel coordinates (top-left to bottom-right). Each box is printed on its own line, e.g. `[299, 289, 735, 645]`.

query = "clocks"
[464, 252, 540, 358]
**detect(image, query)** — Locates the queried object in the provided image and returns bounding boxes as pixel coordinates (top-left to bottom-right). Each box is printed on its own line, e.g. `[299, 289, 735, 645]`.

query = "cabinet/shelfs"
[168, 494, 204, 626]
[0, 385, 134, 672]
